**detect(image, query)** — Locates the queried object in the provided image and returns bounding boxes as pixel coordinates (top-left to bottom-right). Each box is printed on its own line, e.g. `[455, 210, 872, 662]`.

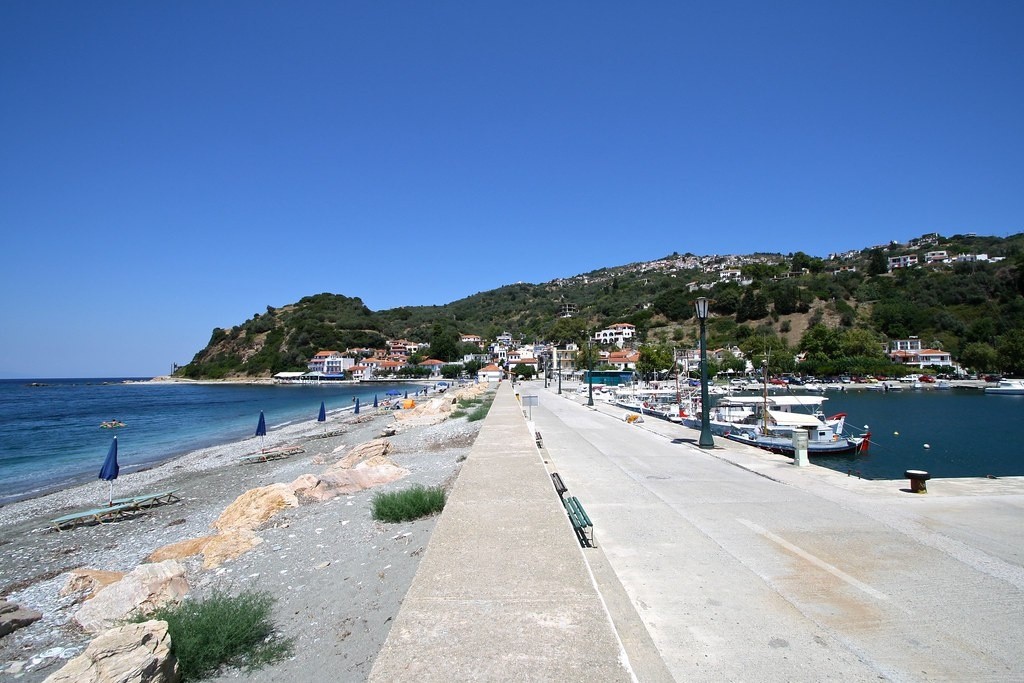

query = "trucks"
[897, 376, 918, 382]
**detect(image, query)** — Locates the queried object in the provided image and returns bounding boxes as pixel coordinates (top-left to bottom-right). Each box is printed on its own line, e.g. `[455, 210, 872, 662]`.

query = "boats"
[984, 380, 1024, 395]
[910, 382, 924, 391]
[100, 422, 128, 429]
[933, 380, 951, 390]
[576, 359, 902, 423]
[681, 394, 865, 455]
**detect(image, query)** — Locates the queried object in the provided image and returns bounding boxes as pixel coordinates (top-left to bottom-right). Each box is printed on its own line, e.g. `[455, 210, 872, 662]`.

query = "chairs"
[293, 390, 423, 441]
[251, 444, 305, 456]
[49, 503, 128, 532]
[237, 450, 289, 466]
[102, 489, 179, 511]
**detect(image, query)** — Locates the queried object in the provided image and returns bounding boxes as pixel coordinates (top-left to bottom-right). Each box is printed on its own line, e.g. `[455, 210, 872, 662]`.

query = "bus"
[583, 369, 635, 386]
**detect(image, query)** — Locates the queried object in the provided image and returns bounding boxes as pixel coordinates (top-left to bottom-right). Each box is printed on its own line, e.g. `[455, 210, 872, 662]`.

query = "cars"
[822, 377, 831, 384]
[919, 376, 935, 383]
[938, 374, 956, 380]
[867, 376, 878, 383]
[830, 377, 839, 383]
[689, 379, 699, 386]
[838, 376, 850, 383]
[790, 378, 805, 385]
[813, 378, 827, 384]
[877, 376, 886, 380]
[851, 375, 857, 380]
[803, 376, 812, 383]
[746, 377, 757, 384]
[730, 379, 749, 386]
[769, 378, 784, 384]
[855, 377, 867, 383]
[708, 379, 714, 386]
[758, 377, 764, 383]
[682, 378, 693, 383]
[779, 377, 789, 384]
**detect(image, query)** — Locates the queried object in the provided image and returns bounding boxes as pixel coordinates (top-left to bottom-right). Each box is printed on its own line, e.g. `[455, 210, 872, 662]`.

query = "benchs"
[535, 432, 542, 440]
[550, 473, 568, 496]
[563, 496, 593, 529]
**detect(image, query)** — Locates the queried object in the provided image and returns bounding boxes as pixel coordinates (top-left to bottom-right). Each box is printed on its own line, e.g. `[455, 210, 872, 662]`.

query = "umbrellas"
[415, 388, 418, 398]
[438, 382, 447, 386]
[373, 394, 378, 412]
[405, 390, 407, 398]
[385, 389, 401, 400]
[318, 401, 326, 431]
[98, 438, 119, 508]
[354, 398, 359, 423]
[255, 412, 266, 453]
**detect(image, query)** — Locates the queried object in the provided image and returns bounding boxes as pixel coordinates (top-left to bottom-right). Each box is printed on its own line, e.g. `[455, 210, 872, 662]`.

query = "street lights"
[542, 355, 550, 387]
[587, 340, 597, 405]
[556, 350, 564, 394]
[687, 297, 717, 448]
